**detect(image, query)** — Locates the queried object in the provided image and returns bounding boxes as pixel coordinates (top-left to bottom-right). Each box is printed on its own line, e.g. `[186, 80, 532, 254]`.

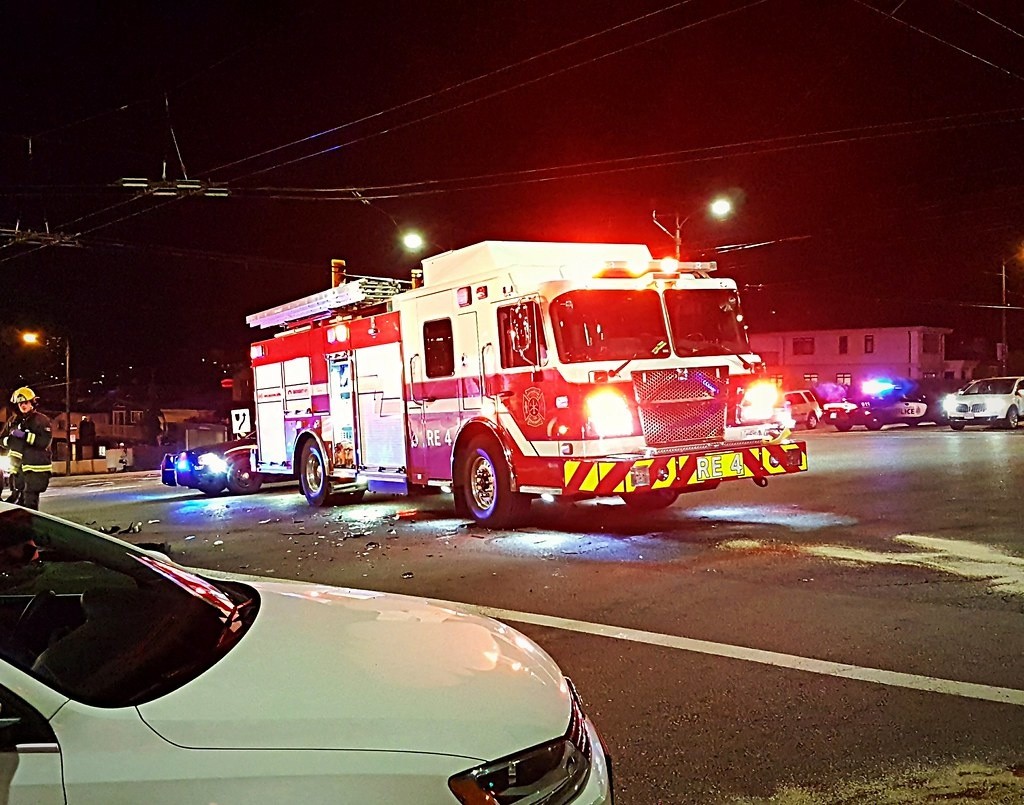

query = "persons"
[121, 448, 128, 472]
[0, 387, 53, 509]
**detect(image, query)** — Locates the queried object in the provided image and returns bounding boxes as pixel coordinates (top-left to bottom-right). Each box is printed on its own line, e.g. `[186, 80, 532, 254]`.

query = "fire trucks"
[244, 240, 809, 532]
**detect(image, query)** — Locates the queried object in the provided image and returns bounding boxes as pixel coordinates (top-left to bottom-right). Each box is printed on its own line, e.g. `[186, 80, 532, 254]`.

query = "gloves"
[12, 429, 25, 438]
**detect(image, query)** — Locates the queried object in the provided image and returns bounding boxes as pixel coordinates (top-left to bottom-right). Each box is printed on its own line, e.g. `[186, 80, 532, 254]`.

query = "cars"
[783, 389, 822, 430]
[942, 376, 1024, 431]
[821, 377, 951, 431]
[159, 430, 302, 498]
[0, 498, 615, 805]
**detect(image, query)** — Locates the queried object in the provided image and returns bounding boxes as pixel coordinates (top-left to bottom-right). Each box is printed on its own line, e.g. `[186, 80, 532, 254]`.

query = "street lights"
[676, 199, 731, 262]
[24, 332, 72, 475]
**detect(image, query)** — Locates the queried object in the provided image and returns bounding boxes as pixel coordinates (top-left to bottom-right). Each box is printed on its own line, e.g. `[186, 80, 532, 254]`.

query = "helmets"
[11, 387, 35, 404]
